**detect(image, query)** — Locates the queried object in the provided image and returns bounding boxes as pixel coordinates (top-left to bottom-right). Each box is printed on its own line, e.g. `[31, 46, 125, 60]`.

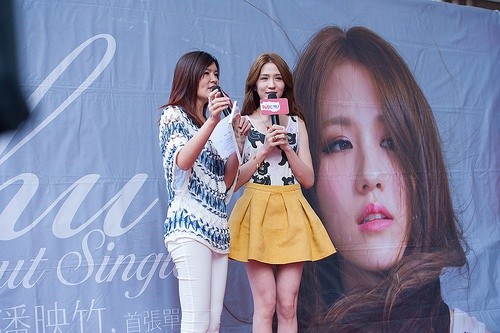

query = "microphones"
[212, 86, 232, 117]
[260, 93, 289, 126]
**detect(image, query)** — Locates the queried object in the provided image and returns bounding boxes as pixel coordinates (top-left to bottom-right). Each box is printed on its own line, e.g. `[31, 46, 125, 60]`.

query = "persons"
[228, 52, 337, 333]
[158, 51, 252, 333]
[223, 25, 492, 333]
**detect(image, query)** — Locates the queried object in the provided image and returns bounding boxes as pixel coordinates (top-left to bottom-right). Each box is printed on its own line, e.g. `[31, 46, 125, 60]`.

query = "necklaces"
[256, 109, 270, 130]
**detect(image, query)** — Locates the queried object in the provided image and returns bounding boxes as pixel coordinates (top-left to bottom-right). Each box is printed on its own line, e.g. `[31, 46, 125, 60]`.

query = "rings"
[272, 136, 277, 141]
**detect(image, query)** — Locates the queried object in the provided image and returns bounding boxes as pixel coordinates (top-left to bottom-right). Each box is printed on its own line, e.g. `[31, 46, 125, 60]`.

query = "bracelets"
[252, 153, 260, 168]
